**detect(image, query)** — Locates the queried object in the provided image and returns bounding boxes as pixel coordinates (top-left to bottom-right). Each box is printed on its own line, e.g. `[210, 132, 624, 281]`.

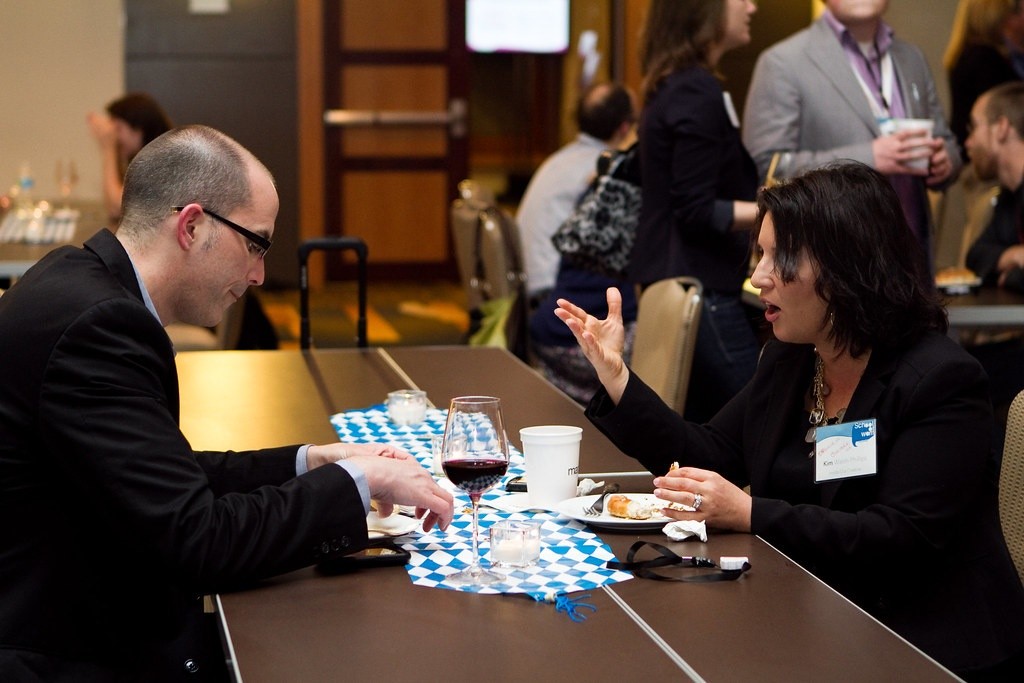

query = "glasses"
[171, 203, 275, 262]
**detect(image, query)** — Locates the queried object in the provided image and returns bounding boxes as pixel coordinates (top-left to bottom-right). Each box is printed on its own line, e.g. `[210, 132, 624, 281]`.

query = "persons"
[0, 94, 456, 683]
[554, 158, 1024, 683]
[516, 0, 1023, 395]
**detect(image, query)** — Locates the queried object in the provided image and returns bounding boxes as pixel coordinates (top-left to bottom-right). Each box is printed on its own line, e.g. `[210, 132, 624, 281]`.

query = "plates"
[367, 511, 419, 538]
[553, 493, 695, 528]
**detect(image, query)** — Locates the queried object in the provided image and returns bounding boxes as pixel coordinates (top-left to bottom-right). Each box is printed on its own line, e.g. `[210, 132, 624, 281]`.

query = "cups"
[432, 435, 466, 475]
[892, 118, 933, 169]
[388, 390, 426, 424]
[520, 426, 583, 507]
[489, 520, 542, 569]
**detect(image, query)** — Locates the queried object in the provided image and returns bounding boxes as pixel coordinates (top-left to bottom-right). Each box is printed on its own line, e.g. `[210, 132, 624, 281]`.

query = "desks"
[0, 193, 110, 284]
[175, 345, 966, 683]
[945, 285, 1024, 338]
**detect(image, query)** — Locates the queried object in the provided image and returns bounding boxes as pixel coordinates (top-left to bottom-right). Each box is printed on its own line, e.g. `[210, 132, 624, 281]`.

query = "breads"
[607, 494, 639, 518]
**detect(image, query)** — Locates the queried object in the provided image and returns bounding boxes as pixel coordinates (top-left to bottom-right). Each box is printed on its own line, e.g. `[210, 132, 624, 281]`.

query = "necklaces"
[805, 348, 848, 444]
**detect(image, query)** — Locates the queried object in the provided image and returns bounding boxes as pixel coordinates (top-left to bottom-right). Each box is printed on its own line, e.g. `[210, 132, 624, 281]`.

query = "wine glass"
[55, 159, 77, 210]
[442, 395, 511, 585]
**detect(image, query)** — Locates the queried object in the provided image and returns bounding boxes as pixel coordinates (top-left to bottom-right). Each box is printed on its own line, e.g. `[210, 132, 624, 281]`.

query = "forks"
[583, 483, 621, 516]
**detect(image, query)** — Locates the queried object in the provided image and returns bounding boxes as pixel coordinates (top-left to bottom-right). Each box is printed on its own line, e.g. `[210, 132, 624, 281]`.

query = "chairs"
[934, 180, 985, 269]
[473, 204, 532, 357]
[628, 277, 703, 418]
[448, 198, 485, 302]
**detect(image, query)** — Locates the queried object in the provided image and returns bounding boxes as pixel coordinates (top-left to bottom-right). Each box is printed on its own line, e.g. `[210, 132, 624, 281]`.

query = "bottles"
[17, 159, 34, 209]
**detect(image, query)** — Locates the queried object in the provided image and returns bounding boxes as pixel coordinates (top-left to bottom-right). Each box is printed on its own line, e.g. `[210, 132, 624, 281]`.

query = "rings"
[692, 493, 703, 509]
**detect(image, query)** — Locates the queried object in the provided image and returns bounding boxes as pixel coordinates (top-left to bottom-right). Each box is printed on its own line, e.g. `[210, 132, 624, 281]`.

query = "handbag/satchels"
[552, 153, 642, 279]
